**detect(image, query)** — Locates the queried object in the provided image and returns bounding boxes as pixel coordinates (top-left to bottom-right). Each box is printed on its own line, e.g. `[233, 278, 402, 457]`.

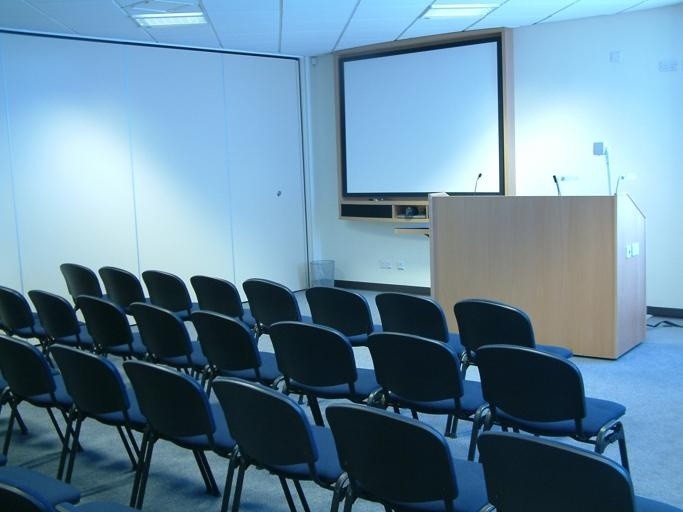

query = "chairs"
[0, 263, 683, 511]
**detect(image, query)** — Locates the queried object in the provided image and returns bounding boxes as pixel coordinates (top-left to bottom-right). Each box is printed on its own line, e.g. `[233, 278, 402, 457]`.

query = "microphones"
[474, 174, 482, 195]
[553, 175, 561, 196]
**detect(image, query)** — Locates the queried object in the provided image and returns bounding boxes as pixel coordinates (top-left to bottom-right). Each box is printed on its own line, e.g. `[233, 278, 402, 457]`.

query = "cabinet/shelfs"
[339, 199, 429, 222]
